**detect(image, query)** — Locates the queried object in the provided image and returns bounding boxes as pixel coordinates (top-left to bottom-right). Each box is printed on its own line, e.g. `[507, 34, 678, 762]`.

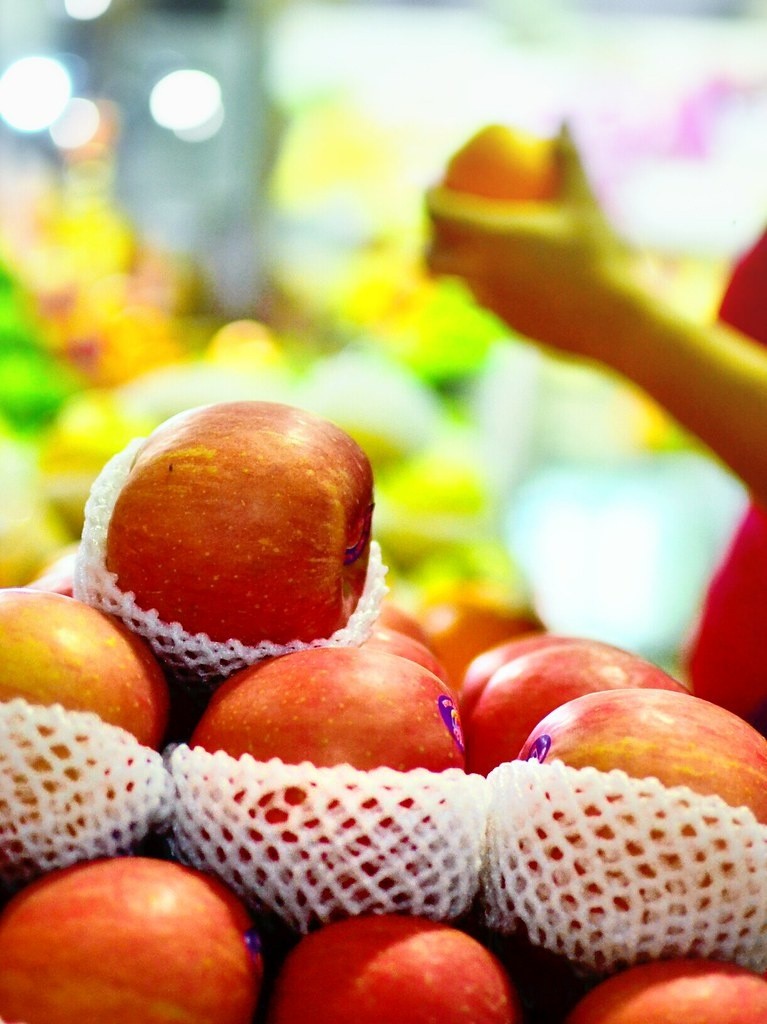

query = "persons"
[428, 120, 765, 720]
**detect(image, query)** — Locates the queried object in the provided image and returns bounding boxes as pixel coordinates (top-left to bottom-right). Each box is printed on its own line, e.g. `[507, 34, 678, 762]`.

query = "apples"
[0, 124, 767, 1024]
